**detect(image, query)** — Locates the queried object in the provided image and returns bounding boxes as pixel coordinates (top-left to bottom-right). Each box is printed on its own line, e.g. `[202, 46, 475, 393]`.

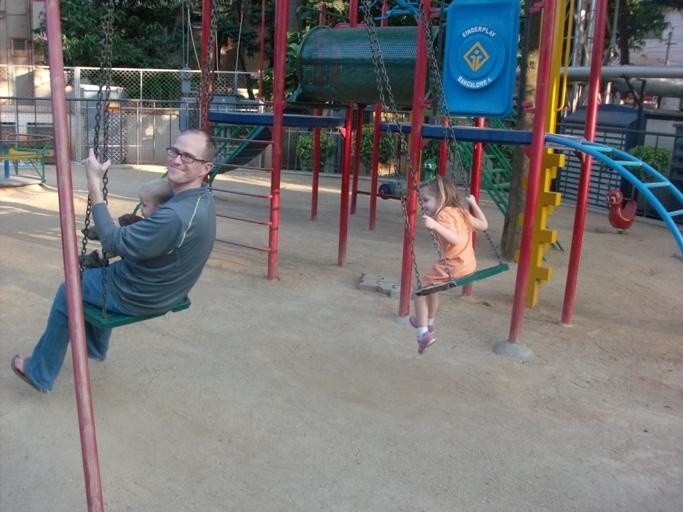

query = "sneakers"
[79, 227, 110, 268]
[416, 330, 436, 355]
[409, 314, 435, 335]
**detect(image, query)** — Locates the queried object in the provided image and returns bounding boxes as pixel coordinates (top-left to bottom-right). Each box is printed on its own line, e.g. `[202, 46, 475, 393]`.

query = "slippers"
[10, 352, 53, 395]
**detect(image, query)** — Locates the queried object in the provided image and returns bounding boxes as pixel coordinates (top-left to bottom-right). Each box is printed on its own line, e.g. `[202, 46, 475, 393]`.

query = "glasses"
[166, 145, 209, 165]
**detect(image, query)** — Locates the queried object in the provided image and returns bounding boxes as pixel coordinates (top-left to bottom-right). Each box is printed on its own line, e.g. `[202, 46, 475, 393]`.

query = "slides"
[210, 83, 300, 174]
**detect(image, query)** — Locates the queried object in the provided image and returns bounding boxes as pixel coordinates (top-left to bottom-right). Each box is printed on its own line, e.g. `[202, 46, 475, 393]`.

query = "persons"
[10, 127, 221, 395]
[76, 178, 173, 269]
[404, 174, 490, 354]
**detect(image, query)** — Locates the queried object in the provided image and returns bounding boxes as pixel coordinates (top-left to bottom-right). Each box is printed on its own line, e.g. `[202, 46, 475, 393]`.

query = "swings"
[358, 1, 508, 296]
[79, 1, 216, 326]
